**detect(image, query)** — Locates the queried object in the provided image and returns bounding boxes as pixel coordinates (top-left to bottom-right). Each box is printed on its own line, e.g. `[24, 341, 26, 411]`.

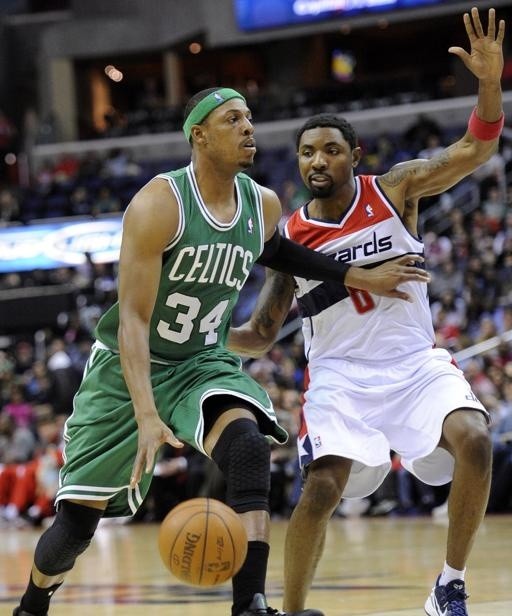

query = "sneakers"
[426, 572, 466, 615]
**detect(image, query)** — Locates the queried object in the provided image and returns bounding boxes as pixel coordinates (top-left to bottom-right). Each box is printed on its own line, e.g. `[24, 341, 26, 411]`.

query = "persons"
[0, 40, 512, 518]
[223, 6, 504, 616]
[12, 87, 431, 614]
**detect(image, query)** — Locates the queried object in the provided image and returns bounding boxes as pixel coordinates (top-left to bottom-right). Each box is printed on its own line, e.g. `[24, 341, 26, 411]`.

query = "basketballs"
[159, 497, 248, 588]
